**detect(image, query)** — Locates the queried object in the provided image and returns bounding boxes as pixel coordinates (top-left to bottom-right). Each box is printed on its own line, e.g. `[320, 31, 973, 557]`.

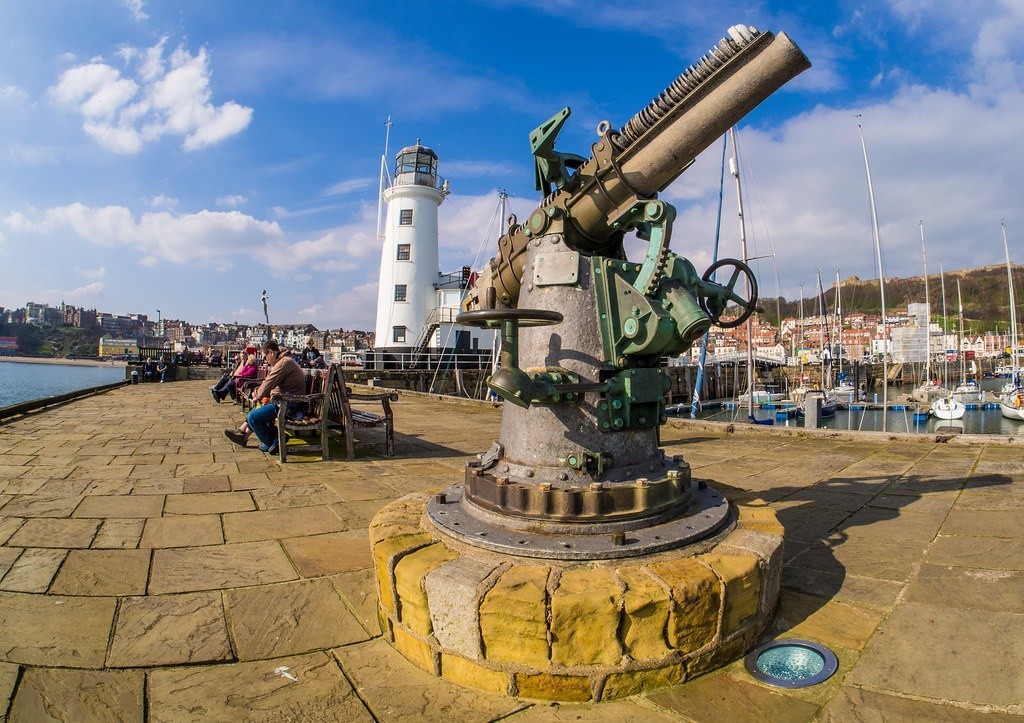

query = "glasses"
[263, 352, 268, 360]
[306, 343, 311, 344]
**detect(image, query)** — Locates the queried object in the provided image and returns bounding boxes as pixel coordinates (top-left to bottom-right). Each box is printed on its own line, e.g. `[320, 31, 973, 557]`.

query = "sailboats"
[738, 114, 1024, 422]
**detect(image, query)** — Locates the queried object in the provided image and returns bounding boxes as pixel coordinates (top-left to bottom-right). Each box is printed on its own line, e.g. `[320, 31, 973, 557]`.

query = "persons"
[157, 356, 167, 384]
[174, 347, 202, 367]
[224, 339, 307, 456]
[303, 338, 325, 369]
[142, 357, 154, 383]
[212, 351, 258, 403]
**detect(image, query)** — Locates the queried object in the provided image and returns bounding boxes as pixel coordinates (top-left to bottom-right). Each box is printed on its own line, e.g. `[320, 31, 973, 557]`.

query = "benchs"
[142, 362, 177, 383]
[220, 361, 399, 464]
[177, 354, 208, 366]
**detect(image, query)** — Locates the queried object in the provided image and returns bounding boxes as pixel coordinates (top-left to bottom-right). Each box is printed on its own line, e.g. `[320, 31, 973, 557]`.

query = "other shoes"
[225, 429, 250, 447]
[269, 433, 290, 454]
[161, 380, 164, 382]
[212, 390, 220, 403]
[258, 443, 270, 450]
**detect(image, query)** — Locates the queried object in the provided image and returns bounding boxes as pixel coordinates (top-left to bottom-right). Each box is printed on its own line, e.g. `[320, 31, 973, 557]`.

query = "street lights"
[157, 309, 160, 347]
